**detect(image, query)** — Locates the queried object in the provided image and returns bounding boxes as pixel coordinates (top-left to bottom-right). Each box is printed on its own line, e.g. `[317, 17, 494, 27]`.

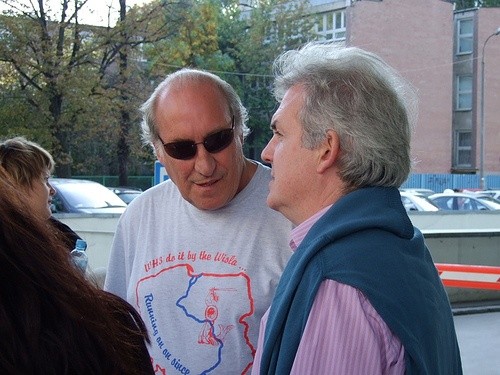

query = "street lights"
[477, 28, 500, 191]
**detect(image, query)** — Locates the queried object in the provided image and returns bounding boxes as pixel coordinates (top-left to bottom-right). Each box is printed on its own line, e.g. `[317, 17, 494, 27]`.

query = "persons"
[102, 68, 297, 375]
[0, 137, 158, 375]
[250, 42, 464, 375]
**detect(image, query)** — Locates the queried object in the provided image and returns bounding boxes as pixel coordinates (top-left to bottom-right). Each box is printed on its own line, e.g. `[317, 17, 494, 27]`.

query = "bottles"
[70, 239, 88, 276]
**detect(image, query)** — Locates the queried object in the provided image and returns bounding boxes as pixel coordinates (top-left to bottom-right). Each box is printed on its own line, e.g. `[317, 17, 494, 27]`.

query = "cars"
[399, 186, 500, 214]
[48, 175, 128, 215]
[107, 187, 143, 205]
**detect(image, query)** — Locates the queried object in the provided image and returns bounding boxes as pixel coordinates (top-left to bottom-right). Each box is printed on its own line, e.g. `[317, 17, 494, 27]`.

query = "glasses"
[158, 115, 235, 160]
